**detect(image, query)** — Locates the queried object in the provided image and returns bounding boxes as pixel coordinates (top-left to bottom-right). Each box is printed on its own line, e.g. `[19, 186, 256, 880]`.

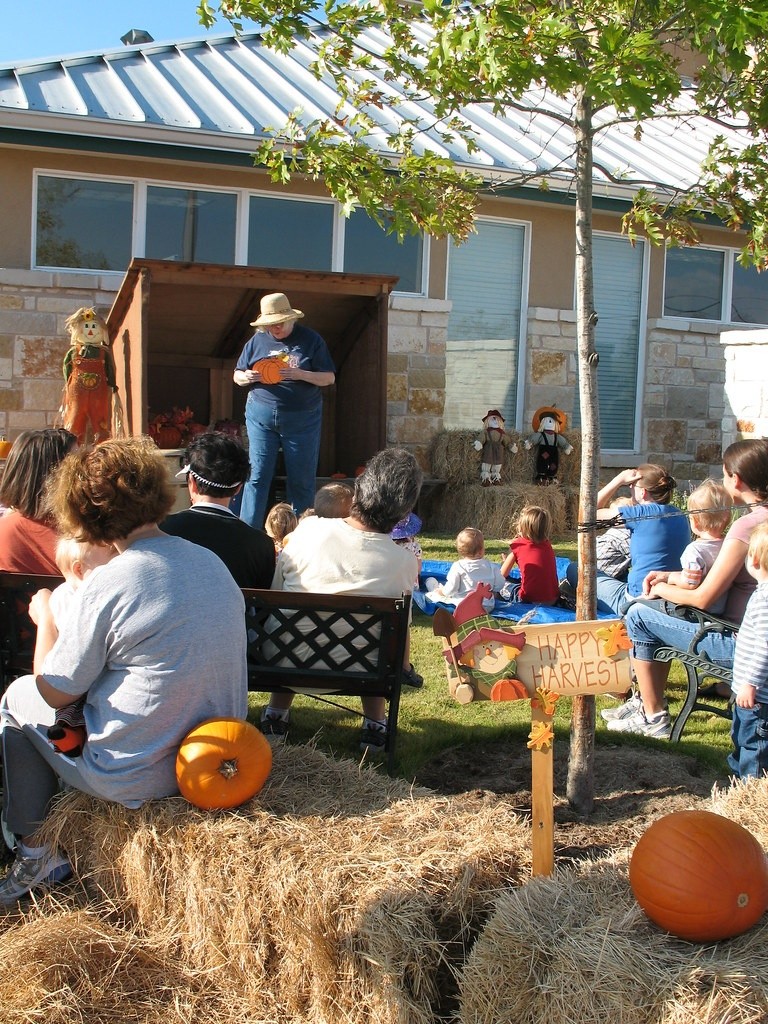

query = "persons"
[63, 307, 118, 448]
[474, 410, 518, 487]
[233, 293, 336, 533]
[523, 406, 573, 487]
[0, 428, 768, 904]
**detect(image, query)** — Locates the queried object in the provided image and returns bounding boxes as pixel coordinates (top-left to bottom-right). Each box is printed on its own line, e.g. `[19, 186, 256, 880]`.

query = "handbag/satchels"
[49, 695, 85, 757]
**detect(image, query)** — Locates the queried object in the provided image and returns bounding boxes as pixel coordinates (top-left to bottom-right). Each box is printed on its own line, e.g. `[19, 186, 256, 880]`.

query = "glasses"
[265, 322, 283, 330]
[629, 483, 643, 489]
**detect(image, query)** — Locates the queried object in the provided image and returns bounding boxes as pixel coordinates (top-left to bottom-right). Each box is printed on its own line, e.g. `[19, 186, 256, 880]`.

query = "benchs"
[0, 571, 412, 746]
[654, 603, 743, 744]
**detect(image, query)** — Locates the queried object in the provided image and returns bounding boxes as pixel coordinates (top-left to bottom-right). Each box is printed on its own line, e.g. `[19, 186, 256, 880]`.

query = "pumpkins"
[330, 470, 346, 477]
[532, 403, 566, 434]
[629, 809, 768, 943]
[152, 426, 182, 449]
[0, 436, 12, 458]
[354, 466, 365, 477]
[175, 716, 272, 810]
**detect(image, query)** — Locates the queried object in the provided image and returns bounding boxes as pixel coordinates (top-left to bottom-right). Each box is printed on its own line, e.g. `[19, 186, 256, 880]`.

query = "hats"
[250, 293, 304, 325]
[391, 512, 422, 539]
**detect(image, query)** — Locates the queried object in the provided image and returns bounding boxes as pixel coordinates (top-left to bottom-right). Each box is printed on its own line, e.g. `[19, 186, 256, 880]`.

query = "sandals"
[401, 663, 423, 687]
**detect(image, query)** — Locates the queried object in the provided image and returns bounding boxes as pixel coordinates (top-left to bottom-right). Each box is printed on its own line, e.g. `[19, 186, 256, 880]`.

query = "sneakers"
[602, 682, 669, 720]
[0, 842, 72, 901]
[260, 703, 288, 748]
[361, 718, 387, 751]
[607, 704, 673, 739]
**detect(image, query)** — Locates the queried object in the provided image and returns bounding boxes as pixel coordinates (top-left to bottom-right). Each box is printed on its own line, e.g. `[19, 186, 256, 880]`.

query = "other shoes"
[426, 577, 439, 591]
